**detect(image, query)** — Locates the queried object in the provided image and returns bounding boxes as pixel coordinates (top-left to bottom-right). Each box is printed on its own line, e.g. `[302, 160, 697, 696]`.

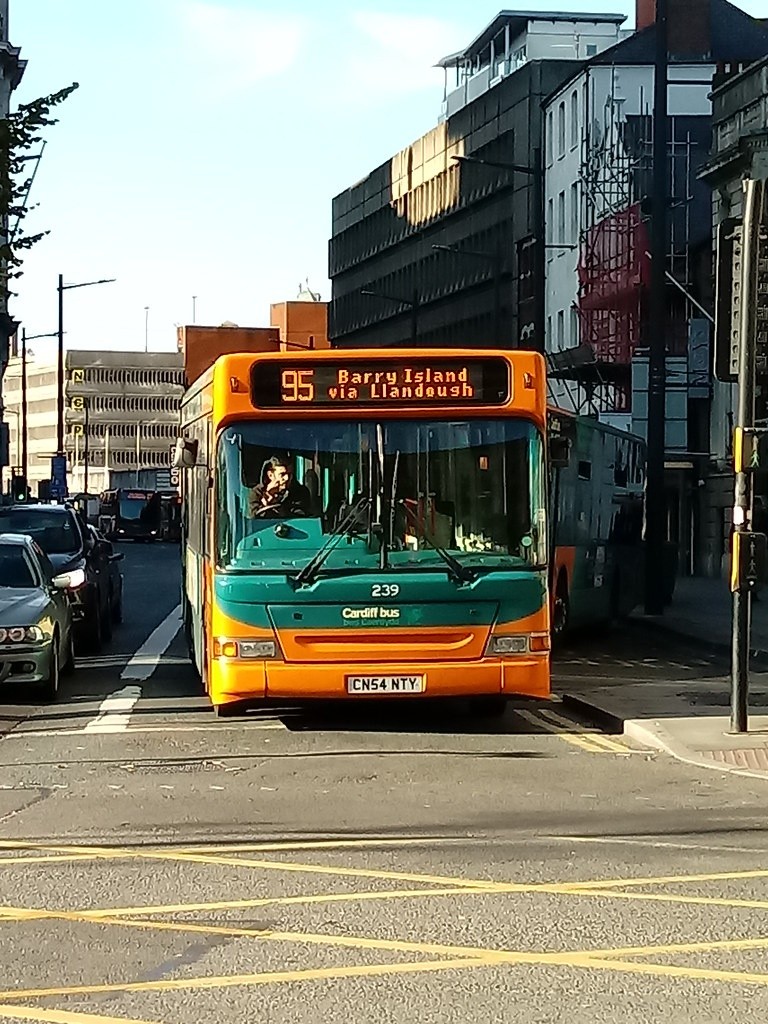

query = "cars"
[0, 504, 125, 640]
[0, 533, 74, 692]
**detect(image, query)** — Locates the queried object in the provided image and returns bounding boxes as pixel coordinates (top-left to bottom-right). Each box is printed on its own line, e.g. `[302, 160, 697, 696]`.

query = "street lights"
[21, 328, 66, 478]
[358, 288, 420, 349]
[450, 148, 545, 353]
[59, 274, 116, 457]
[433, 243, 505, 346]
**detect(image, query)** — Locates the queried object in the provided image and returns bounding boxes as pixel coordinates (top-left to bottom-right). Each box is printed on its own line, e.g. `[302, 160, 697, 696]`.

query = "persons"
[250, 459, 310, 519]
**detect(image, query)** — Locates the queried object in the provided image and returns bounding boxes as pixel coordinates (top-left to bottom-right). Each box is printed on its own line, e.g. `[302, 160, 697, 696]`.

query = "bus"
[98, 489, 162, 542]
[174, 349, 645, 717]
